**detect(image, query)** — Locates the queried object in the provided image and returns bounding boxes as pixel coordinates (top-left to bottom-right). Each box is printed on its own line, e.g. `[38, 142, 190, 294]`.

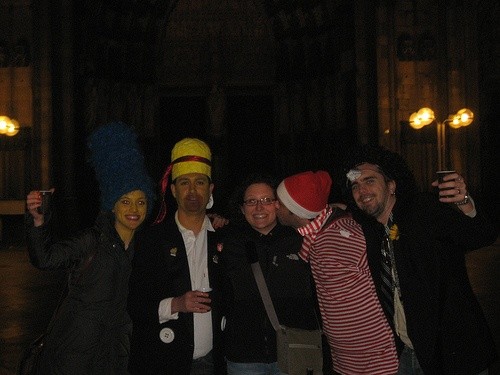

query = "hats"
[153, 138, 212, 224]
[276, 171, 332, 219]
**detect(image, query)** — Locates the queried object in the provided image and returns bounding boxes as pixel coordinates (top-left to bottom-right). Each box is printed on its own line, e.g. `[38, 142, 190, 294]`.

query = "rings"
[458, 188, 461, 194]
[456, 175, 461, 180]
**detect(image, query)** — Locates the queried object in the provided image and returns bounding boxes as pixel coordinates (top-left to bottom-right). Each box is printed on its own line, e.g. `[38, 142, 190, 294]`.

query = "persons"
[211, 173, 315, 375]
[127, 137, 234, 375]
[331, 146, 500, 375]
[273, 170, 399, 375]
[24, 121, 230, 374]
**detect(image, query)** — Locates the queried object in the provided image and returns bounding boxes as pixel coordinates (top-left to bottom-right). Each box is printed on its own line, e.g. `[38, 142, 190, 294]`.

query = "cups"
[436, 170, 457, 199]
[195, 288, 212, 313]
[39, 191, 51, 209]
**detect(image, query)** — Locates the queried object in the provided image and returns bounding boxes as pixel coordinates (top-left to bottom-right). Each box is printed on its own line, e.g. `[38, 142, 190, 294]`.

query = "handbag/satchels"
[276, 325, 324, 375]
[16, 332, 47, 375]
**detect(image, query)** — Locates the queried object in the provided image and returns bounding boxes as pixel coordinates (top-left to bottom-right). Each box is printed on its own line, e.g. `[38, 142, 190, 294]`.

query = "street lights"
[408, 107, 474, 172]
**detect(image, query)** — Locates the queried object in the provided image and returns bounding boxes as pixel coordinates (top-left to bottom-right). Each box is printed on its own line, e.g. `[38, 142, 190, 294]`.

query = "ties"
[379, 211, 404, 321]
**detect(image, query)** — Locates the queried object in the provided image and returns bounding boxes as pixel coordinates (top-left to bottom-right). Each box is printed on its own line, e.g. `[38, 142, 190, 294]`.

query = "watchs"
[453, 192, 470, 206]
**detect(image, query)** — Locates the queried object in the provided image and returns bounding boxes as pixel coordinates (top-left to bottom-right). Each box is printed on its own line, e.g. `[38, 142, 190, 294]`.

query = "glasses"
[242, 198, 276, 206]
